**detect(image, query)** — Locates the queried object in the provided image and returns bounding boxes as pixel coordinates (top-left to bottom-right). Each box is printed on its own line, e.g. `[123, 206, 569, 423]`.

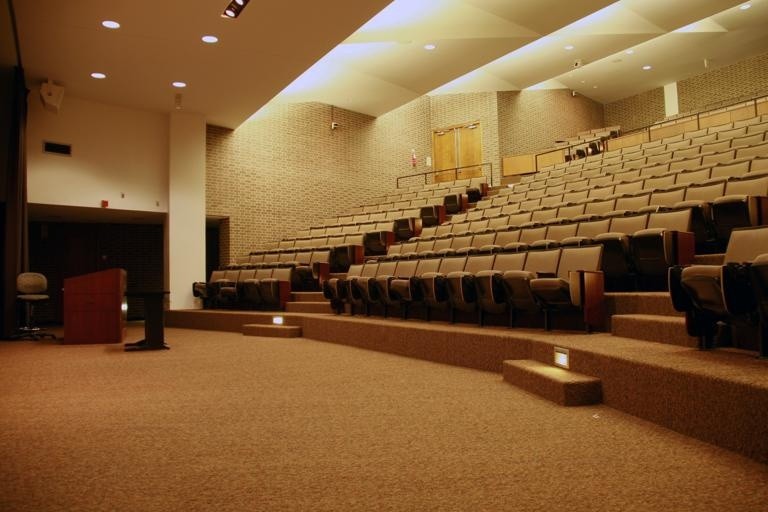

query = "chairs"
[190, 115, 768, 315]
[383, 258, 442, 320]
[667, 228, 768, 340]
[474, 251, 526, 330]
[503, 247, 562, 331]
[446, 254, 494, 325]
[11, 271, 57, 341]
[526, 244, 605, 332]
[410, 255, 467, 322]
